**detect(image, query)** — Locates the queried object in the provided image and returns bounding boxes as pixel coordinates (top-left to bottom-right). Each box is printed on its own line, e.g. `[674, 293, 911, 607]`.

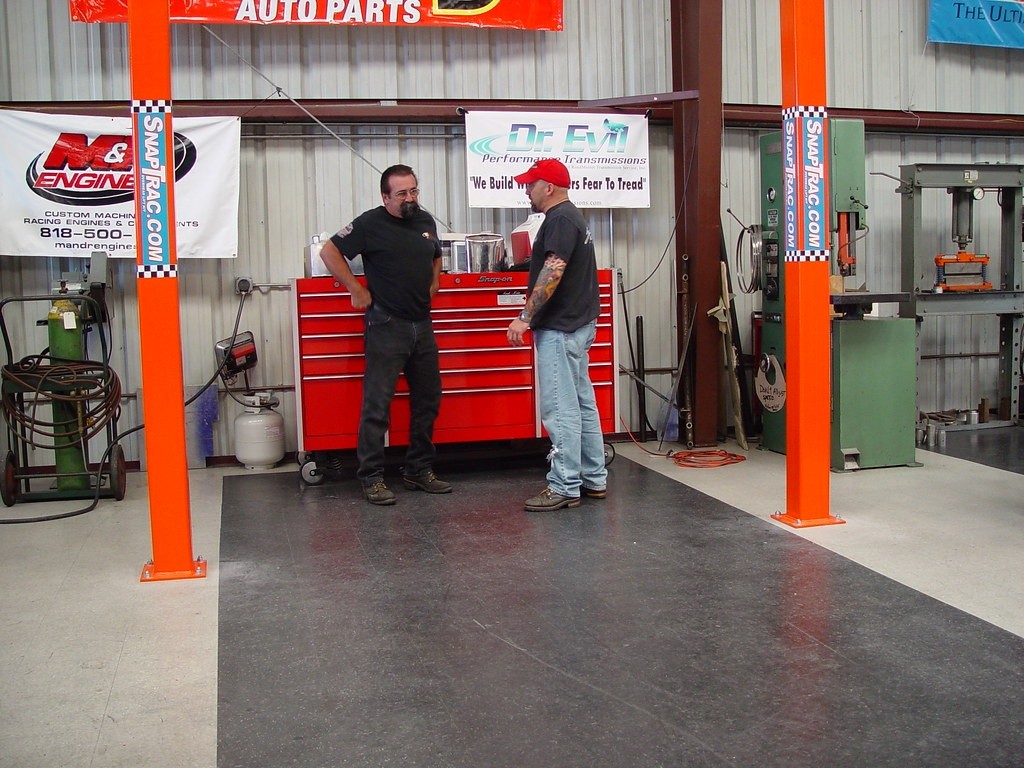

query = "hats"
[513, 159, 570, 190]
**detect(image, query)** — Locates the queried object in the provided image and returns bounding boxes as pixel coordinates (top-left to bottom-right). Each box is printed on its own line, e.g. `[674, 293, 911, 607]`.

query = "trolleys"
[0, 293, 127, 507]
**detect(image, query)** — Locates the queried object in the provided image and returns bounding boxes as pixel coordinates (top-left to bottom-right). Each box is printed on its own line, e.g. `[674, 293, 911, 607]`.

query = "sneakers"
[402, 467, 452, 494]
[360, 479, 397, 505]
[581, 485, 608, 498]
[523, 487, 581, 511]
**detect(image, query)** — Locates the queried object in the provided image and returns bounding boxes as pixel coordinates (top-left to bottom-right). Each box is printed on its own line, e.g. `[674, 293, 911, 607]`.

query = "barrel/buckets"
[465, 233, 505, 273]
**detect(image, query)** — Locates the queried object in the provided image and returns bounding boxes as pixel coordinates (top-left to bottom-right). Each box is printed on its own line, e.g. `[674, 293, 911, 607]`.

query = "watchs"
[520, 312, 531, 323]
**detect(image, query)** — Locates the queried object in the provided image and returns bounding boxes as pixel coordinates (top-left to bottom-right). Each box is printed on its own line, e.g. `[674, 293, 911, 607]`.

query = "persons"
[507, 160, 608, 511]
[320, 165, 453, 505]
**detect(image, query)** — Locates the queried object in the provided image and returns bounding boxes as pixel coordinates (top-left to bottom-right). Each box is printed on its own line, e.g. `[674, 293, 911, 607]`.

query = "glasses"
[385, 188, 420, 199]
